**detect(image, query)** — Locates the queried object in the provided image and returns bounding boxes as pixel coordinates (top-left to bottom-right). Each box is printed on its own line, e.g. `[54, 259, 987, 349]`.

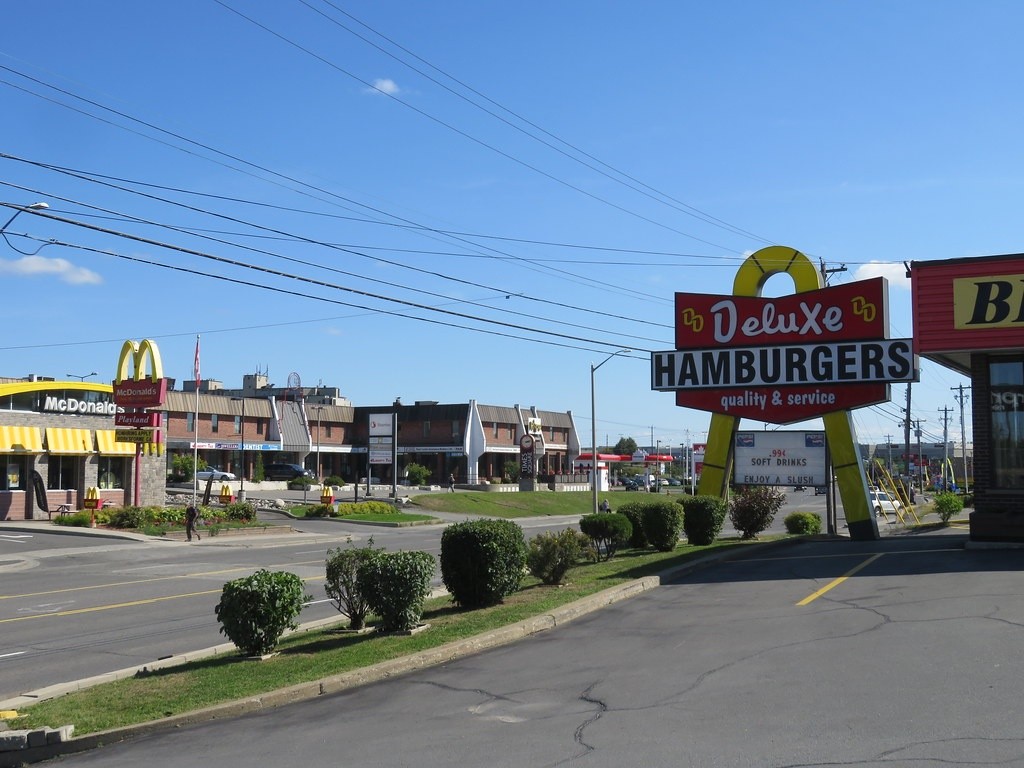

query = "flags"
[194, 341, 200, 388]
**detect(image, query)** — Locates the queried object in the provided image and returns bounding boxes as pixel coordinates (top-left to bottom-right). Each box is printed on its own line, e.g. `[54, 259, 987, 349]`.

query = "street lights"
[647, 443, 685, 490]
[312, 406, 326, 481]
[231, 397, 246, 503]
[590, 348, 632, 515]
[66, 372, 98, 382]
[656, 440, 661, 491]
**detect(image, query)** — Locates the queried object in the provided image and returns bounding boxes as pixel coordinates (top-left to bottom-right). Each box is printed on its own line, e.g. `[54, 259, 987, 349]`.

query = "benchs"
[49, 509, 67, 513]
[61, 511, 78, 513]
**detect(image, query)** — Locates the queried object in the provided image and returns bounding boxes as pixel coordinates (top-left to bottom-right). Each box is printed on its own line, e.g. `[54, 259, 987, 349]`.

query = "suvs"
[869, 486, 901, 517]
[897, 486, 916, 505]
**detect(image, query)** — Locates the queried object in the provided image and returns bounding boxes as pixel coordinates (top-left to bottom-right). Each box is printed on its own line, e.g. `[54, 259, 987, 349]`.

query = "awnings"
[0, 426, 137, 457]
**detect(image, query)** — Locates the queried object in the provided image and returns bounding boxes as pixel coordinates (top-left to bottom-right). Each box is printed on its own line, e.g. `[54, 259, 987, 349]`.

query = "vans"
[264, 462, 311, 481]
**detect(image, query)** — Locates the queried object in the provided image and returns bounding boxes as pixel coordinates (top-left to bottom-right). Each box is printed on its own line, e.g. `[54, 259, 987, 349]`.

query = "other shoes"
[198, 533, 201, 541]
[185, 537, 191, 541]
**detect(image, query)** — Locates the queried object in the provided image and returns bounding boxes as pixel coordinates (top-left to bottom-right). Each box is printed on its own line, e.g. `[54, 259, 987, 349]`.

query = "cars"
[794, 486, 807, 492]
[196, 465, 236, 481]
[618, 474, 681, 491]
[815, 486, 827, 496]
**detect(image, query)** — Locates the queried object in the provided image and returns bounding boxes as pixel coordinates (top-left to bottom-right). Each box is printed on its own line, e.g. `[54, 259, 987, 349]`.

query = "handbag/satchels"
[182, 518, 188, 525]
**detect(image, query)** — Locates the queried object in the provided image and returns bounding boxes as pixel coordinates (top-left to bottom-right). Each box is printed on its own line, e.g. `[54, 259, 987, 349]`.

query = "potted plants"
[323, 476, 345, 491]
[287, 477, 311, 491]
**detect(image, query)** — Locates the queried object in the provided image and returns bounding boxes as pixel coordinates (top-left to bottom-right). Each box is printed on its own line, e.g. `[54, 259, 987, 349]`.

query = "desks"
[102, 503, 116, 508]
[54, 504, 73, 517]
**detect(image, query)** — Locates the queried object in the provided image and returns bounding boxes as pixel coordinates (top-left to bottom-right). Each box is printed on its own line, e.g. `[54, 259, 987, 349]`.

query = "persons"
[947, 482, 953, 492]
[185, 502, 200, 542]
[602, 499, 609, 513]
[448, 473, 455, 492]
